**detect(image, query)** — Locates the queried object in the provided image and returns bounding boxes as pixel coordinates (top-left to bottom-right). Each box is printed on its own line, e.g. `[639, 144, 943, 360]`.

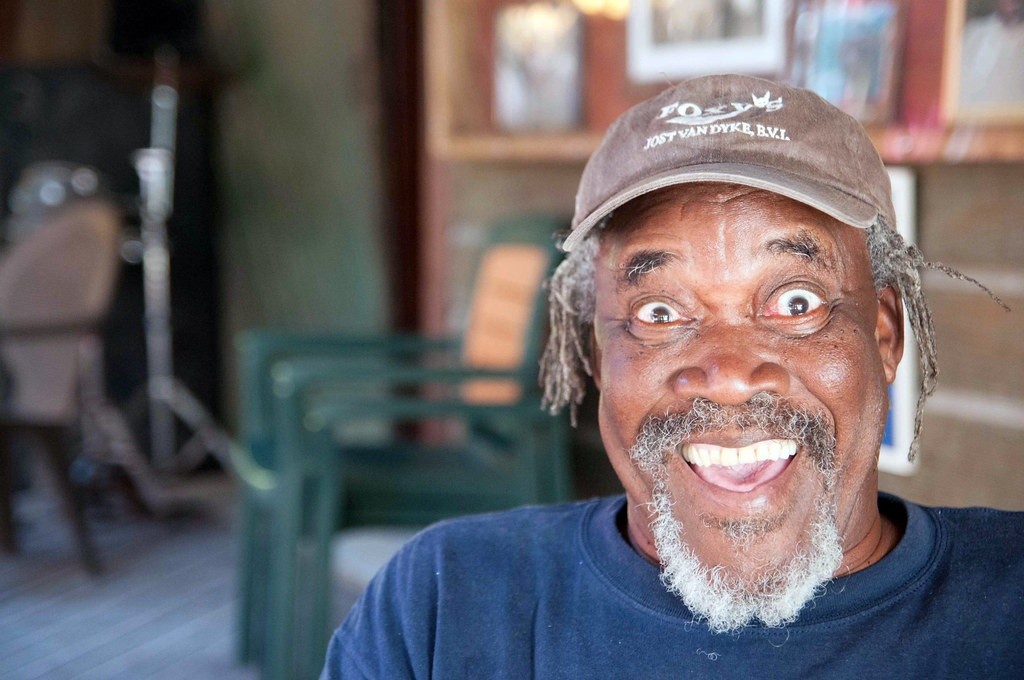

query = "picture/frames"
[624, 0, 786, 83]
[938, 0, 1024, 132]
[876, 163, 918, 476]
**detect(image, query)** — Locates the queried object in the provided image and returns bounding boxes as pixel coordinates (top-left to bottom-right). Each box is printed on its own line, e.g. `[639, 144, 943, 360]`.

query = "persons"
[315, 73, 1024, 680]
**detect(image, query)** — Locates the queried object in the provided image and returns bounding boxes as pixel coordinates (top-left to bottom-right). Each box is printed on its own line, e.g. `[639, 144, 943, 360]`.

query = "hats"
[559, 72, 898, 235]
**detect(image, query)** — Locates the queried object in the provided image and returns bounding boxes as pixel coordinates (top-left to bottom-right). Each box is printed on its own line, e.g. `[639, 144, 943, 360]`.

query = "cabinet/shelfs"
[413, 0, 1024, 514]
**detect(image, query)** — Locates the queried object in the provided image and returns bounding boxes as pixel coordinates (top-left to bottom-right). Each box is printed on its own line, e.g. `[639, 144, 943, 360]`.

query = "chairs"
[0, 200, 123, 570]
[237, 213, 592, 679]
